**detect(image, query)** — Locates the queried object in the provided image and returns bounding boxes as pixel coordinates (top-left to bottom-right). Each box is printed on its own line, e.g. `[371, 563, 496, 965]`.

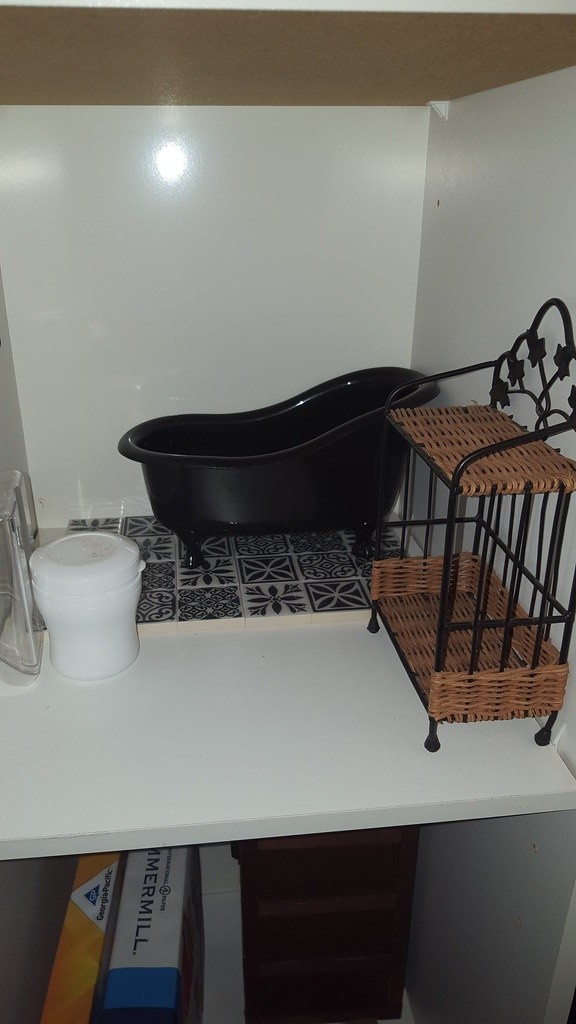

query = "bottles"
[28, 531, 147, 682]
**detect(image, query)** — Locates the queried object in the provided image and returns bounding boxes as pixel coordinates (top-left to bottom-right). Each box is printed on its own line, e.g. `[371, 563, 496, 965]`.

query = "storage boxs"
[42, 846, 204, 1024]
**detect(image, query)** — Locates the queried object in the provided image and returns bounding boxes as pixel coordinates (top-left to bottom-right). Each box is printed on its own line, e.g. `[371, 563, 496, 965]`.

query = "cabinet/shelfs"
[367, 305, 576, 751]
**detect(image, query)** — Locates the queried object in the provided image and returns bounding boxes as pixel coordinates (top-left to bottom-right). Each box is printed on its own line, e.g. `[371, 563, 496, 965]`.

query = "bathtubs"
[115, 363, 443, 570]
